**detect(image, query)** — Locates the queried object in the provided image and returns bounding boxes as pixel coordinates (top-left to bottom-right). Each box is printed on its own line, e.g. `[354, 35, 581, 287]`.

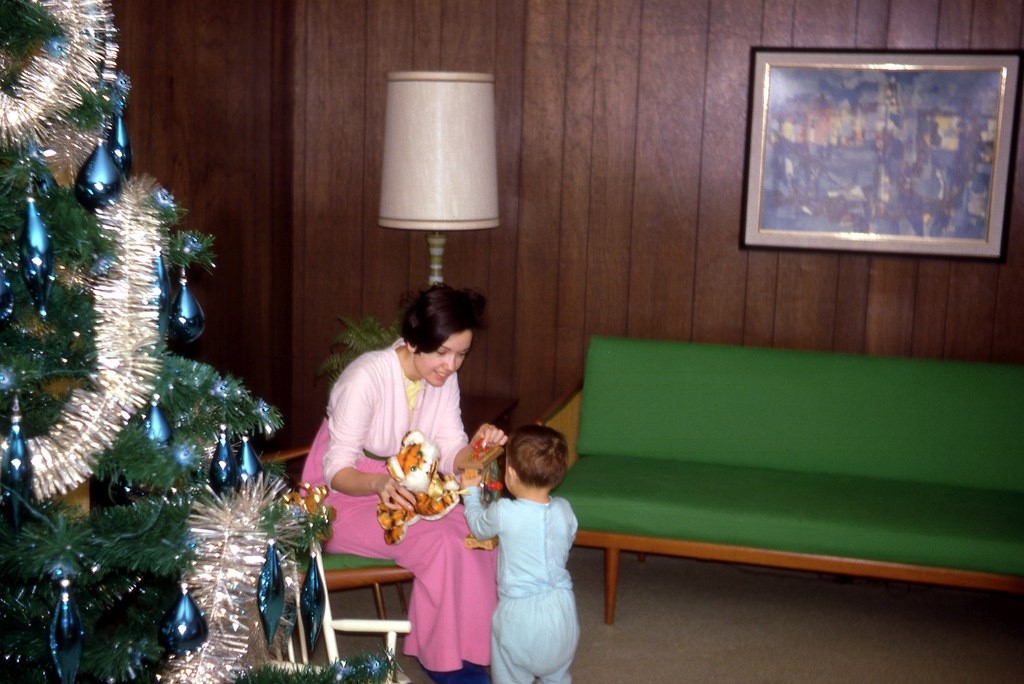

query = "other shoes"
[417, 657, 492, 684]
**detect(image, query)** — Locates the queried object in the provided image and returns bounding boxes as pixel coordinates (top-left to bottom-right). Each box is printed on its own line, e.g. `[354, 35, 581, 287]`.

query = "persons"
[299, 282, 508, 684]
[461, 425, 580, 684]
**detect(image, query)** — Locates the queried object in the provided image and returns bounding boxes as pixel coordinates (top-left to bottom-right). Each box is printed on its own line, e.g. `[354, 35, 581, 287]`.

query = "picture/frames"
[739, 45, 1022, 263]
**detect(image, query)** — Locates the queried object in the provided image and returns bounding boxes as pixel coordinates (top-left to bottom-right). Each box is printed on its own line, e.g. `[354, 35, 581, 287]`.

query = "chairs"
[268, 540, 412, 684]
[262, 447, 416, 614]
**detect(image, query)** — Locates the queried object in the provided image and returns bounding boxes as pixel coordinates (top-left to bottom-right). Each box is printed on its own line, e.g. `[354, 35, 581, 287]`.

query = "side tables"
[459, 393, 519, 479]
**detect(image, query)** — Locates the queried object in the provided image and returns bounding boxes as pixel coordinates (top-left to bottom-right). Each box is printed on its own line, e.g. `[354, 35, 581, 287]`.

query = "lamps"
[379, 66, 502, 281]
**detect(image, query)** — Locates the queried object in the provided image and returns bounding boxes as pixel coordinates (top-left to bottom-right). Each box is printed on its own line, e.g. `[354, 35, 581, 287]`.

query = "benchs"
[539, 334, 1024, 627]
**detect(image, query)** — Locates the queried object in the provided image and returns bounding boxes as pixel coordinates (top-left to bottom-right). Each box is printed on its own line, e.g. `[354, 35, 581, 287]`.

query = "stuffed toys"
[377, 430, 460, 545]
